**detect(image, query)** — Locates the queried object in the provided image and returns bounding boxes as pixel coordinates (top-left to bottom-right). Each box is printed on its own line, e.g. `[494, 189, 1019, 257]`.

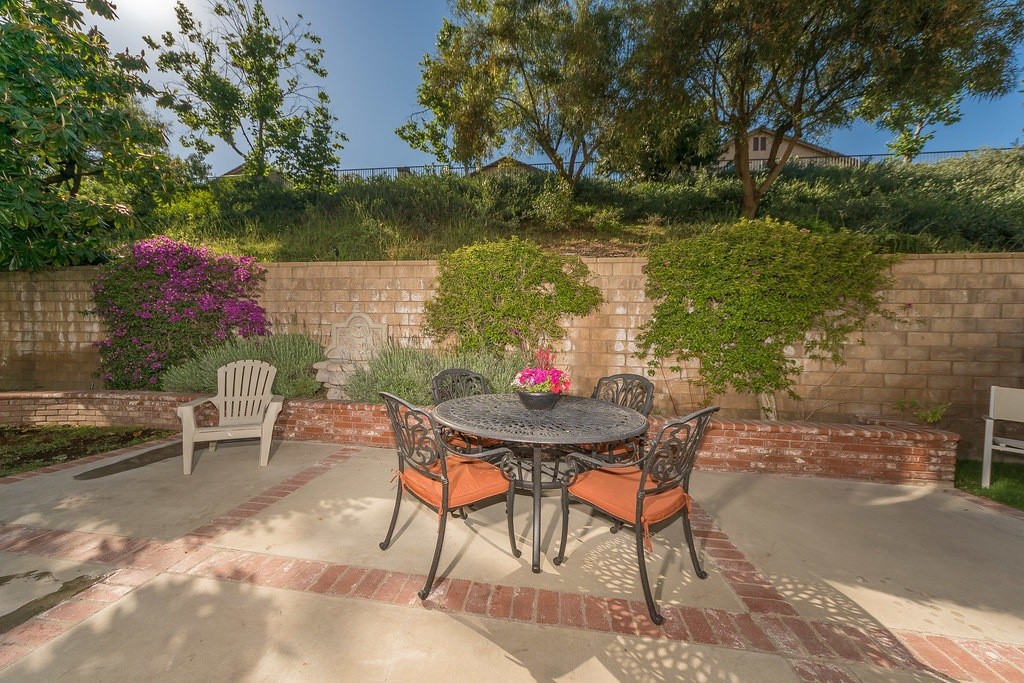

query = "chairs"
[177, 359, 284, 475]
[377, 391, 522, 600]
[562, 372, 655, 526]
[981, 386, 1024, 489]
[554, 403, 719, 625]
[431, 367, 523, 519]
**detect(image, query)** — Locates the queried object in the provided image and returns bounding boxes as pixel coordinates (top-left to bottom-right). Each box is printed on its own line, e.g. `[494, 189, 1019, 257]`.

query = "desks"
[433, 392, 648, 573]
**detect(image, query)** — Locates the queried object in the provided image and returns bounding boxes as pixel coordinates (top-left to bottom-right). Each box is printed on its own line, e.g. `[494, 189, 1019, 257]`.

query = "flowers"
[511, 366, 572, 394]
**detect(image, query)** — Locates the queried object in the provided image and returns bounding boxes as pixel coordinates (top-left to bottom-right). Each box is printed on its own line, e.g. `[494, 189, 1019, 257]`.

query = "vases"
[517, 390, 560, 410]
